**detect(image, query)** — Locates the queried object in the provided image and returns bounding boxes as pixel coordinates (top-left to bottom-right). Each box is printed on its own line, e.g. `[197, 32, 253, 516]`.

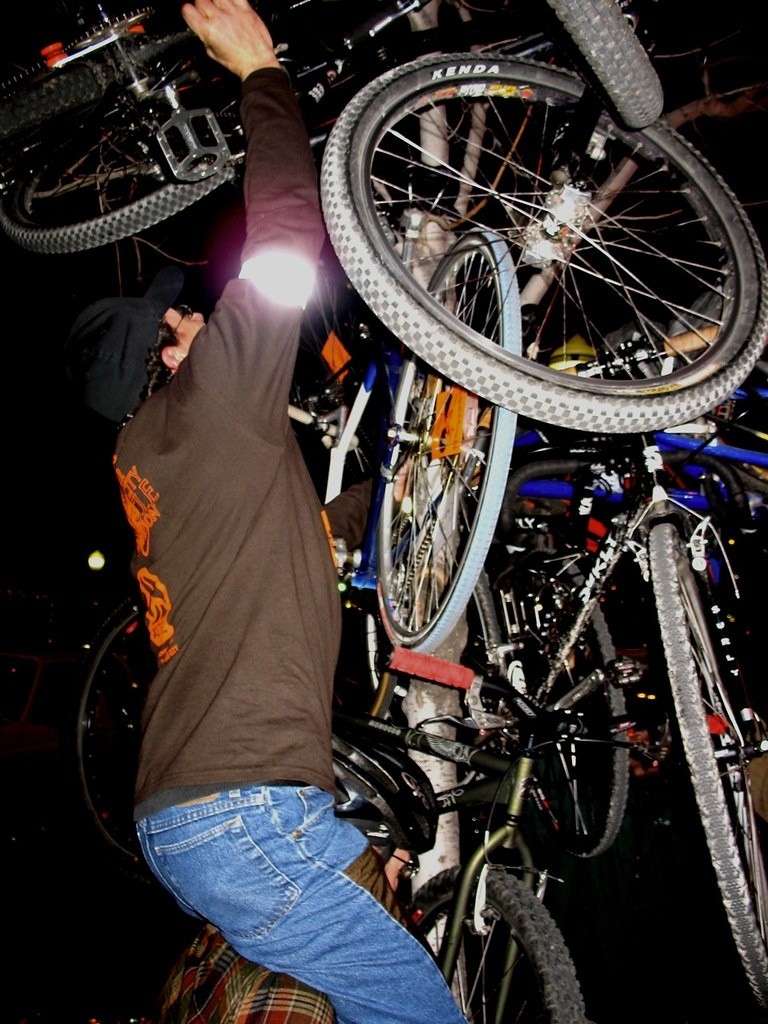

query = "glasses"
[385, 851, 415, 879]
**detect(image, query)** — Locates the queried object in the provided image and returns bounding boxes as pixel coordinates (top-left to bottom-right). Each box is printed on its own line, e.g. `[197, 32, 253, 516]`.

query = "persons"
[58, 0, 482, 1024]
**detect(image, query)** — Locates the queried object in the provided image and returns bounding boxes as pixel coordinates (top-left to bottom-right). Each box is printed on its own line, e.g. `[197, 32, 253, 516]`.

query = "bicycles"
[2, 0, 765, 1023]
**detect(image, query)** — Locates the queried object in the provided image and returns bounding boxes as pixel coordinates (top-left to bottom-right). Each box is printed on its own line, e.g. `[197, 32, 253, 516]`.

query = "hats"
[72, 267, 186, 423]
